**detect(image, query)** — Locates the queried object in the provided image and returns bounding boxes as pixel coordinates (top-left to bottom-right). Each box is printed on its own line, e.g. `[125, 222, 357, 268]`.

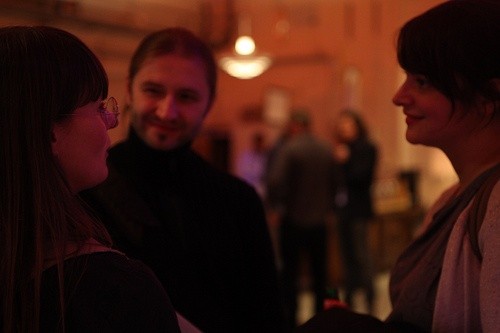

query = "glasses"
[62, 97, 118, 128]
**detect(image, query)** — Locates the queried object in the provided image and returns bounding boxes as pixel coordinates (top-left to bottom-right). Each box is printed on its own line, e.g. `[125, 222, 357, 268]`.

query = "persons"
[388, 1, 500, 333]
[0, 27, 183, 332]
[268, 111, 331, 312]
[318, 108, 378, 313]
[80, 28, 292, 333]
[207, 133, 233, 168]
[236, 133, 271, 200]
